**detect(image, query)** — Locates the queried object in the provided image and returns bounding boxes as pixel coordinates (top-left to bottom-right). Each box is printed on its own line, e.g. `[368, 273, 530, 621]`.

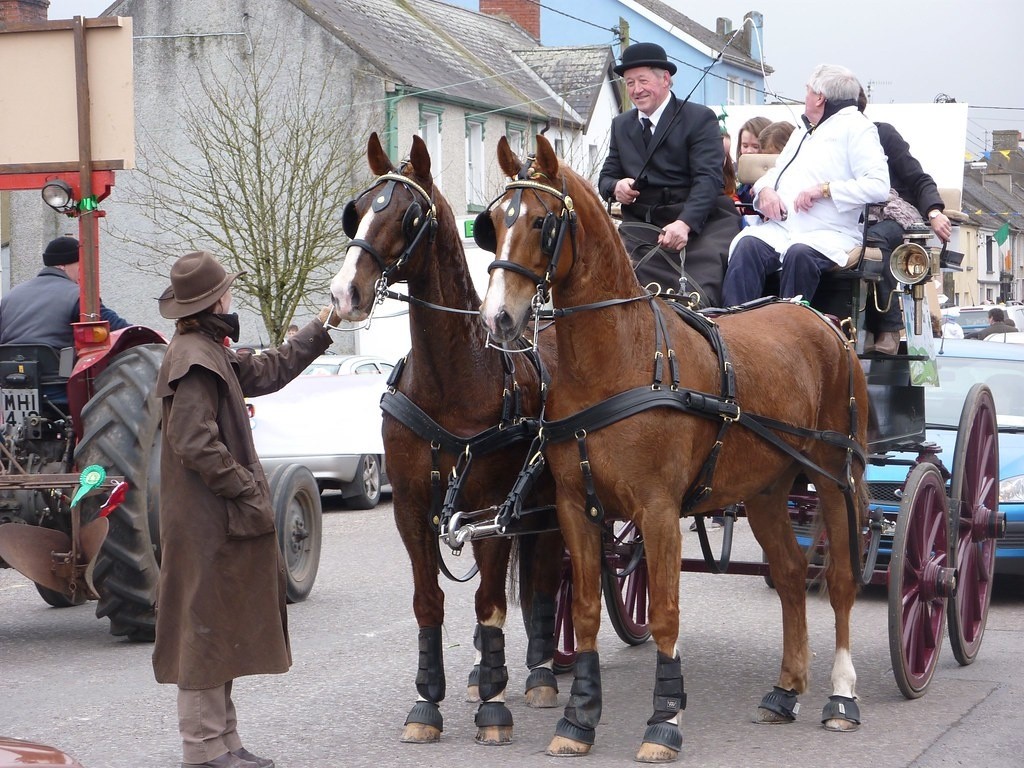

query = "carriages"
[330, 130, 1008, 764]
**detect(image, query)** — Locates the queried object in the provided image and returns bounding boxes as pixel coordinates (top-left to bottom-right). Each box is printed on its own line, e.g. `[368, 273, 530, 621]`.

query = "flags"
[993, 222, 1011, 271]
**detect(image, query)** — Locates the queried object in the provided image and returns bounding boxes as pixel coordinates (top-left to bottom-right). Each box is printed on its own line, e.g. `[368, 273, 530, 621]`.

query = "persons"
[0, 237, 134, 369]
[597, 43, 741, 310]
[719, 64, 951, 355]
[151, 251, 341, 768]
[978, 309, 1019, 341]
[288, 324, 298, 338]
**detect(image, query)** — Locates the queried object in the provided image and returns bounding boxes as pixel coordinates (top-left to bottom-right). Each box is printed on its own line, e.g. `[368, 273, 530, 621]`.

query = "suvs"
[940, 298, 1023, 341]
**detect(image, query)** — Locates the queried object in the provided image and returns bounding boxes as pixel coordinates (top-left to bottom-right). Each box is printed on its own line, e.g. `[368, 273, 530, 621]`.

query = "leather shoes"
[182, 746, 275, 768]
[863, 329, 900, 355]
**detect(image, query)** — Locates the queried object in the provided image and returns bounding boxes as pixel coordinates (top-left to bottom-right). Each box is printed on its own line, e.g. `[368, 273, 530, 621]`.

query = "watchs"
[929, 210, 940, 222]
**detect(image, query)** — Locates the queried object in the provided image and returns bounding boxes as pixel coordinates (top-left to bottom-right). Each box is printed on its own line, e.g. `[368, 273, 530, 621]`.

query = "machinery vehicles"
[0, 322, 324, 643]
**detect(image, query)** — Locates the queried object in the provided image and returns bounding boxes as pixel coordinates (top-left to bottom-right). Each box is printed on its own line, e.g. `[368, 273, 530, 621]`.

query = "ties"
[642, 118, 652, 149]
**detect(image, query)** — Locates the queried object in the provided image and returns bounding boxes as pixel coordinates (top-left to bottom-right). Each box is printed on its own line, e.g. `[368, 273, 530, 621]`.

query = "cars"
[214, 351, 403, 510]
[759, 332, 1024, 590]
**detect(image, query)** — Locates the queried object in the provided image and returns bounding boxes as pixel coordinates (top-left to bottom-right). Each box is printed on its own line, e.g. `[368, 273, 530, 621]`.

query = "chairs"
[986, 373, 1024, 416]
[0, 342, 67, 400]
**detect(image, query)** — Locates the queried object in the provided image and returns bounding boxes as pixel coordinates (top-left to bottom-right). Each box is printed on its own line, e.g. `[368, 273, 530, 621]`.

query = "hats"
[613, 43, 678, 77]
[158, 250, 247, 320]
[42, 236, 79, 266]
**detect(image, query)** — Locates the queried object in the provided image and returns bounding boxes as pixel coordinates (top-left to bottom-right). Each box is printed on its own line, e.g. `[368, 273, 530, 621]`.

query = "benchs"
[925, 185, 969, 228]
[607, 155, 886, 283]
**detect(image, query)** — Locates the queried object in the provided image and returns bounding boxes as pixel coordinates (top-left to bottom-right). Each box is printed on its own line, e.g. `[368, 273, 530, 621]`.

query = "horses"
[326, 131, 879, 764]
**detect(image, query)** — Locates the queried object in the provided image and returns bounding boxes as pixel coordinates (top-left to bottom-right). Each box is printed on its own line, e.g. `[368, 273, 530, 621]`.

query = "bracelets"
[823, 182, 830, 198]
